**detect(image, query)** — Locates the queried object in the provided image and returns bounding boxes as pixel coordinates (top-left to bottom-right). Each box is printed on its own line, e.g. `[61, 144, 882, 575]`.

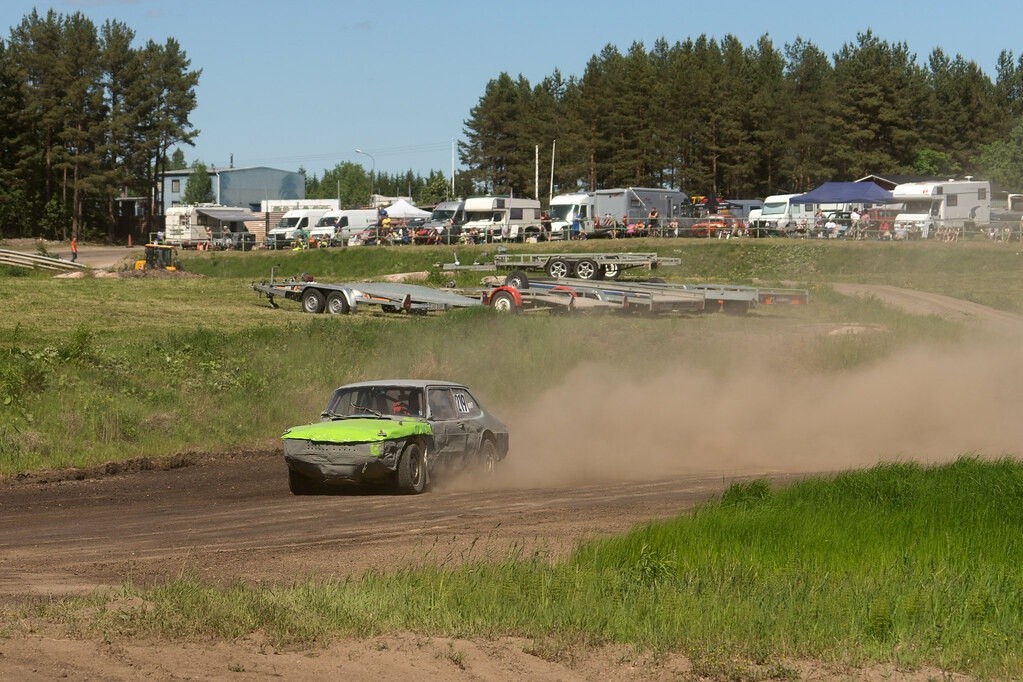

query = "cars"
[691, 214, 747, 239]
[279, 378, 509, 495]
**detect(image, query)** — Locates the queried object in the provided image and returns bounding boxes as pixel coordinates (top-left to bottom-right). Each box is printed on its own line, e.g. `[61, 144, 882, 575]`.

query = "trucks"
[722, 190, 904, 239]
[413, 201, 465, 246]
[305, 209, 378, 248]
[892, 175, 991, 239]
[460, 196, 541, 245]
[595, 186, 700, 237]
[548, 192, 595, 240]
[263, 209, 331, 251]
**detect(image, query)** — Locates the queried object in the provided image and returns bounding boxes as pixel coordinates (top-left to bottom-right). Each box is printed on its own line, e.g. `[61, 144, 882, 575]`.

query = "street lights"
[355, 148, 374, 209]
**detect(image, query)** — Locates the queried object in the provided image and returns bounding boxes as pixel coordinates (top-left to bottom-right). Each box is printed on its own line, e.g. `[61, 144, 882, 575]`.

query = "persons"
[981, 223, 1013, 243]
[382, 215, 442, 245]
[627, 207, 678, 238]
[292, 226, 310, 249]
[459, 227, 480, 245]
[69, 238, 81, 262]
[317, 233, 330, 250]
[795, 207, 959, 241]
[541, 210, 553, 242]
[571, 212, 588, 241]
[391, 391, 421, 417]
[204, 226, 214, 242]
[220, 225, 236, 250]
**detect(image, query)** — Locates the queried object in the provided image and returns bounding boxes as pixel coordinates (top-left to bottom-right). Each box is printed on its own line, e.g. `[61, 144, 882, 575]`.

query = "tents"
[790, 182, 894, 244]
[379, 199, 434, 221]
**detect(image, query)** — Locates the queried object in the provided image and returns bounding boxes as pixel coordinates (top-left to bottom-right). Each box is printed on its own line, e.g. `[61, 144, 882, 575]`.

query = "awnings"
[196, 209, 271, 253]
